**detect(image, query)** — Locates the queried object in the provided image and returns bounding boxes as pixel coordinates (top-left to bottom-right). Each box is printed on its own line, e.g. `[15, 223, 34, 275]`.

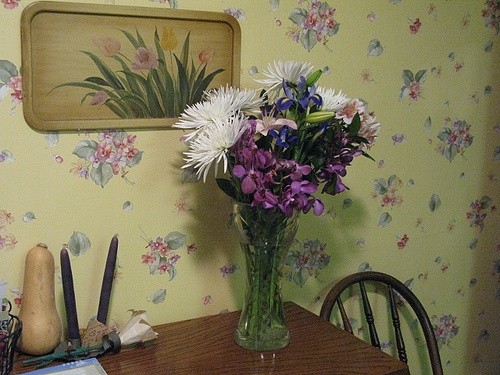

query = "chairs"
[320, 272, 444, 374]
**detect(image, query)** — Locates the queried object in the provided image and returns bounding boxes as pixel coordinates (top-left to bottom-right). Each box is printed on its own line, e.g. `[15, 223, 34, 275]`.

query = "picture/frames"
[20, 2, 241, 130]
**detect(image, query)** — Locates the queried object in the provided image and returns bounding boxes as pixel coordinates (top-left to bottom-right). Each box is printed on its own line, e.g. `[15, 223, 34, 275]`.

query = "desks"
[0, 301, 410, 375]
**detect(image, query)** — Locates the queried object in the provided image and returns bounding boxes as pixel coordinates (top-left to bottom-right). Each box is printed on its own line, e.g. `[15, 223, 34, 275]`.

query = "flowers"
[171, 61, 381, 204]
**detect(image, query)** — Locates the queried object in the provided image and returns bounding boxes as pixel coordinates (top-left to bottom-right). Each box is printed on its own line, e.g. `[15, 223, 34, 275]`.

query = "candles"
[97, 232, 120, 325]
[60, 243, 81, 339]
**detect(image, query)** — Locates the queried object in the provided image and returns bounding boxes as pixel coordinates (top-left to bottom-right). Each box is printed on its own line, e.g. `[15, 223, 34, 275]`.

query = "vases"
[232, 202, 302, 350]
